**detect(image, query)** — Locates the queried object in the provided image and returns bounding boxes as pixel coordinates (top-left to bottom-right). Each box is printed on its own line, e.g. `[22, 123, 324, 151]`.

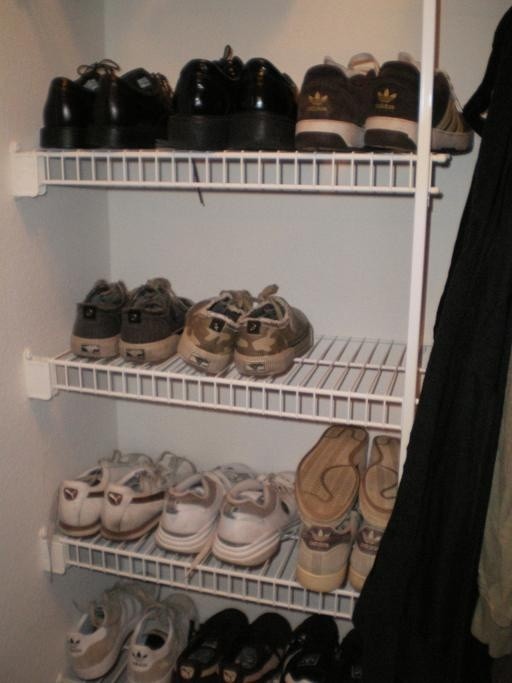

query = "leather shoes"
[40, 45, 297, 151]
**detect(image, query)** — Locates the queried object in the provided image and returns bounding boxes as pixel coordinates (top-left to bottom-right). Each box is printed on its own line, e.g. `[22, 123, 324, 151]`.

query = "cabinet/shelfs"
[17, 4, 436, 681]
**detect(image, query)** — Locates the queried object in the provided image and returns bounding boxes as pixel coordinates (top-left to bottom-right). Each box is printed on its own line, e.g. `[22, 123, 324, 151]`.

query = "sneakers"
[211, 468, 301, 566]
[296, 429, 399, 593]
[294, 60, 474, 153]
[58, 451, 154, 538]
[153, 462, 255, 556]
[101, 452, 198, 541]
[70, 278, 313, 378]
[67, 571, 365, 683]
